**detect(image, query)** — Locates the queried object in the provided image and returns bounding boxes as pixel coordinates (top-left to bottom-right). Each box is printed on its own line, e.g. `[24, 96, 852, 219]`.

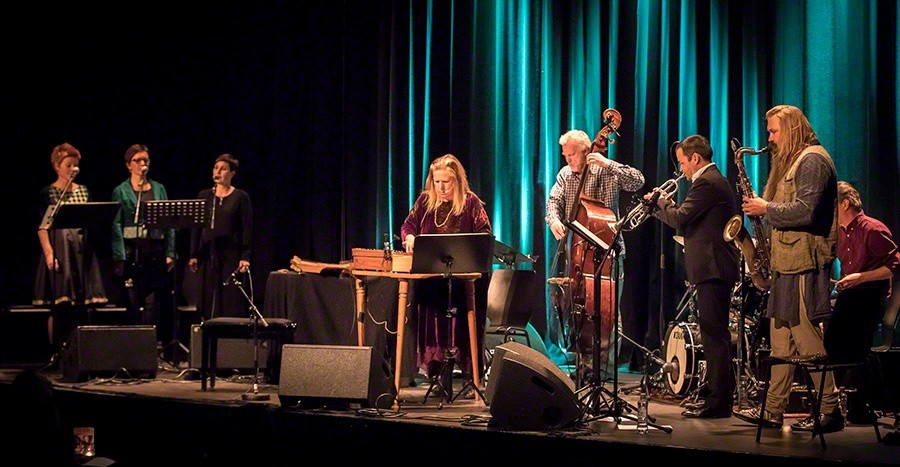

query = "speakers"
[190, 325, 272, 377]
[486, 270, 536, 330]
[278, 345, 397, 411]
[484, 342, 582, 432]
[62, 324, 158, 380]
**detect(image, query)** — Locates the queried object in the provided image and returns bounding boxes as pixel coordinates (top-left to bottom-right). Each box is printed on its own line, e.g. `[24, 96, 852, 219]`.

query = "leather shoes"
[691, 404, 705, 410]
[682, 407, 733, 418]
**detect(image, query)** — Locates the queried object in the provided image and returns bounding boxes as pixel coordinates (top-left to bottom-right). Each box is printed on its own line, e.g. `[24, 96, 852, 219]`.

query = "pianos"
[350, 246, 484, 403]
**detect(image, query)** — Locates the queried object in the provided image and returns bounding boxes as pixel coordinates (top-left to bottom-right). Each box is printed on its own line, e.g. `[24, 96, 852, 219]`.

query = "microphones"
[213, 176, 219, 182]
[663, 362, 678, 373]
[224, 267, 241, 287]
[141, 166, 148, 174]
[641, 192, 661, 221]
[72, 167, 79, 178]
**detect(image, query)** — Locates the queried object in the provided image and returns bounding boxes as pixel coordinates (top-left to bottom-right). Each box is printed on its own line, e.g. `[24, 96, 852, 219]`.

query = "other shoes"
[630, 362, 652, 373]
[463, 389, 475, 399]
[428, 386, 452, 397]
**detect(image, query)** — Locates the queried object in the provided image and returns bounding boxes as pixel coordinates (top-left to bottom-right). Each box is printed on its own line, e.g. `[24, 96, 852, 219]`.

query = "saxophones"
[722, 143, 776, 291]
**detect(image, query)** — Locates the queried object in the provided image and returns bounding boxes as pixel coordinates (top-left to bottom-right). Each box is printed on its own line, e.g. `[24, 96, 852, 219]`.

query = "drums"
[728, 272, 768, 326]
[663, 318, 752, 397]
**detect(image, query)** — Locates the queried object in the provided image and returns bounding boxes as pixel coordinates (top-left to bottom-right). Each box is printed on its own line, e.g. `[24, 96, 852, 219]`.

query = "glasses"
[130, 158, 150, 165]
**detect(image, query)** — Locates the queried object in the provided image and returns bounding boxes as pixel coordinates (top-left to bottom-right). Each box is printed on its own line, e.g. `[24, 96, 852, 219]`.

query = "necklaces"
[434, 206, 454, 227]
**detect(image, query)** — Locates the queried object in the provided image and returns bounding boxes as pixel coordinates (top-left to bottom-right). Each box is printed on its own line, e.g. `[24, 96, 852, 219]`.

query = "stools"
[201, 317, 300, 393]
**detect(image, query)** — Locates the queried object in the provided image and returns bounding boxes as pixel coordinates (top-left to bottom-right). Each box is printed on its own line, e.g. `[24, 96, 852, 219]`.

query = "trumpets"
[606, 172, 686, 237]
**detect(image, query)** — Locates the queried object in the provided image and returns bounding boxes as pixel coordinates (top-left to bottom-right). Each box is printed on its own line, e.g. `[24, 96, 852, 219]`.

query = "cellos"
[560, 108, 624, 390]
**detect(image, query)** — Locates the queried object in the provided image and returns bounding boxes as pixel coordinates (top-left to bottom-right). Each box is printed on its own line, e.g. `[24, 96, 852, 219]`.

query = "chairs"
[463, 269, 536, 382]
[755, 265, 900, 451]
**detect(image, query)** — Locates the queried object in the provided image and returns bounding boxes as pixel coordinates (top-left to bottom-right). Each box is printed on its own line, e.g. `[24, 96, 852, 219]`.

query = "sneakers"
[570, 365, 592, 379]
[587, 370, 614, 382]
[737, 407, 783, 428]
[791, 412, 844, 434]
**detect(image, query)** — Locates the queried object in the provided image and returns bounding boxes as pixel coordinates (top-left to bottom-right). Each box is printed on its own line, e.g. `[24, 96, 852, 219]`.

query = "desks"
[350, 271, 482, 411]
[263, 270, 418, 386]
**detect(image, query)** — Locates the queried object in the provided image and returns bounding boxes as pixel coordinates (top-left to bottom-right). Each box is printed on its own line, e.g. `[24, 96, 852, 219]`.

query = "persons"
[735, 105, 845, 433]
[33, 142, 104, 349]
[641, 136, 740, 418]
[111, 145, 177, 367]
[825, 181, 900, 423]
[188, 153, 253, 322]
[545, 130, 645, 382]
[401, 155, 492, 399]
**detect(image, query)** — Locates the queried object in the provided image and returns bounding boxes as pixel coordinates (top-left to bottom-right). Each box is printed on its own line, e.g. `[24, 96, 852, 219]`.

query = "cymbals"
[672, 235, 685, 246]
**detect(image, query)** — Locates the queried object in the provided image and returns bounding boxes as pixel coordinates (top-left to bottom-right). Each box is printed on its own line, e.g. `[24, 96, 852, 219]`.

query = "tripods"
[571, 193, 674, 434]
[732, 252, 760, 412]
[410, 232, 496, 410]
